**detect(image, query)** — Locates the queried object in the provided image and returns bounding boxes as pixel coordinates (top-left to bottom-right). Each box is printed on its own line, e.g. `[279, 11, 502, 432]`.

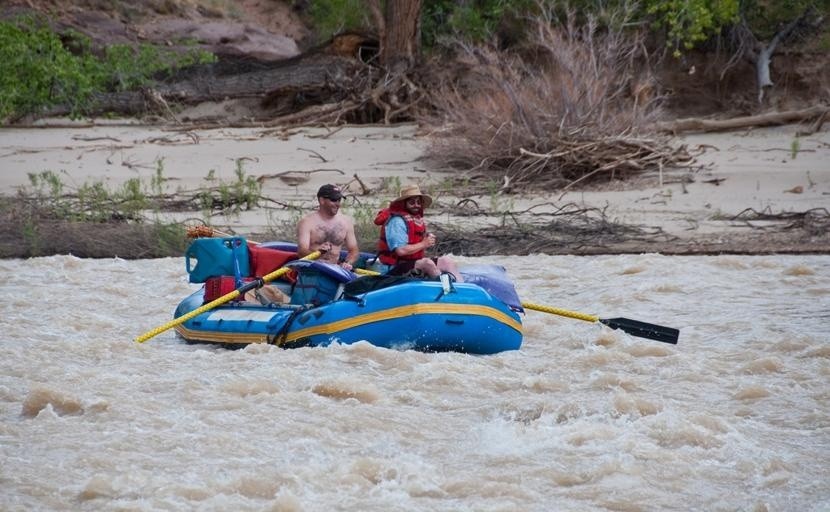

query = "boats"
[176, 236, 524, 355]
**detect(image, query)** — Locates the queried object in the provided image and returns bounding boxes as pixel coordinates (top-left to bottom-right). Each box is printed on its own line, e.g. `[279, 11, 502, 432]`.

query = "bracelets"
[343, 260, 354, 265]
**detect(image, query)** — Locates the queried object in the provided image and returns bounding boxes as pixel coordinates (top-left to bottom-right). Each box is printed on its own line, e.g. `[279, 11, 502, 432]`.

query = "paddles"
[353, 267, 679, 345]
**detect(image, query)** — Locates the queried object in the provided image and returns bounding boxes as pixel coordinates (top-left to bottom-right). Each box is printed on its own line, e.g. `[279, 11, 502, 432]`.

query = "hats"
[317, 184, 346, 200]
[390, 185, 432, 209]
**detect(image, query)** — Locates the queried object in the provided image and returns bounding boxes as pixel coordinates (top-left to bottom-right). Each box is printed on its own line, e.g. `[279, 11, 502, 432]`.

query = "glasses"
[323, 196, 341, 202]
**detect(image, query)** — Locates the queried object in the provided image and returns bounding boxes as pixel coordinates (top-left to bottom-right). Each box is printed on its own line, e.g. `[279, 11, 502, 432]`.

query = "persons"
[290, 183, 360, 271]
[373, 184, 465, 284]
